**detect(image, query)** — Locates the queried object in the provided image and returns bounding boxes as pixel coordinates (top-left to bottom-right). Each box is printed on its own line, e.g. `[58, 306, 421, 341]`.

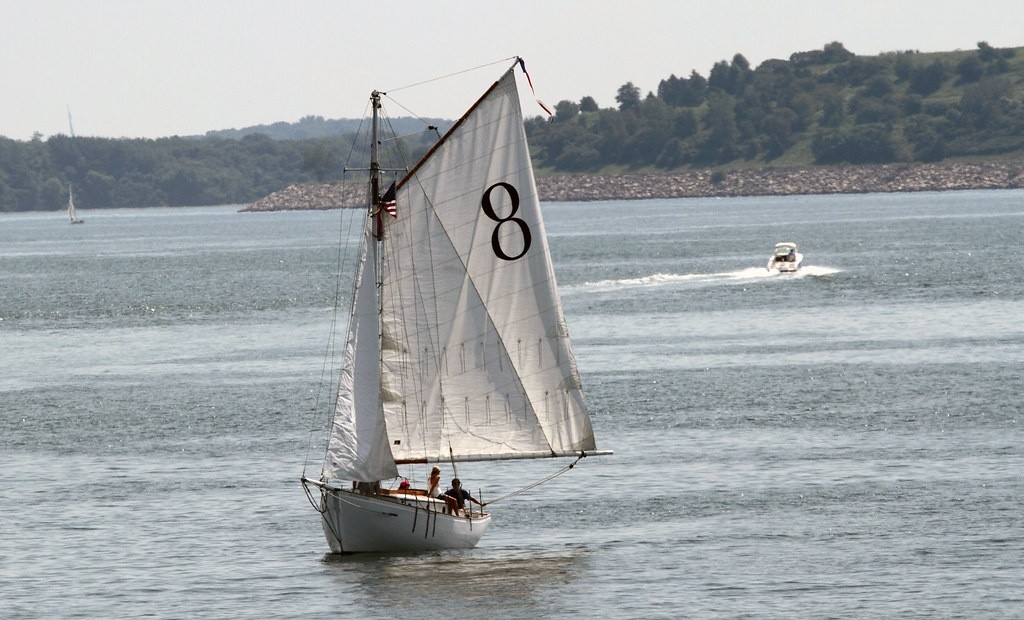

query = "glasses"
[438, 471, 440, 472]
[454, 483, 460, 485]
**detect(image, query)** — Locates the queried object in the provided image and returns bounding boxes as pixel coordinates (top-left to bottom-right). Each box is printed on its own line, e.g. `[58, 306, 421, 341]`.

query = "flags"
[381, 180, 398, 217]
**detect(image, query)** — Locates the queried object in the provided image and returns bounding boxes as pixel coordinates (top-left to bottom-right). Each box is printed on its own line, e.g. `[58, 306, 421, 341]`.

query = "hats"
[452, 479, 461, 486]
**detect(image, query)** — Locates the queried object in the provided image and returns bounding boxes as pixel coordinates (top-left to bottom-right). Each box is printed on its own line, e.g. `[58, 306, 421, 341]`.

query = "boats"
[767, 243, 803, 274]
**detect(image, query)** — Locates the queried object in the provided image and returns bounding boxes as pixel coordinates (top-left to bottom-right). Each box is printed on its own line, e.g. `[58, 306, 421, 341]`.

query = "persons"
[445, 478, 484, 510]
[427, 466, 459, 516]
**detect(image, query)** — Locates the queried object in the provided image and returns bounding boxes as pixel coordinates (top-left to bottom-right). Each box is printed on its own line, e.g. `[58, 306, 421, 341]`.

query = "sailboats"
[299, 49, 611, 559]
[67, 186, 86, 225]
